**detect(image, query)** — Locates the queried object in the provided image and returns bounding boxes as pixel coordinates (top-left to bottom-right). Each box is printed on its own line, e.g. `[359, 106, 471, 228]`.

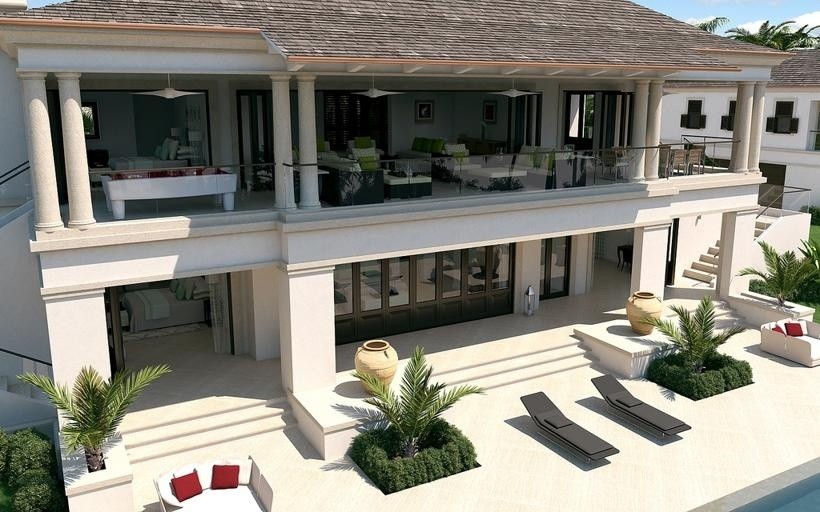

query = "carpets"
[121, 322, 209, 343]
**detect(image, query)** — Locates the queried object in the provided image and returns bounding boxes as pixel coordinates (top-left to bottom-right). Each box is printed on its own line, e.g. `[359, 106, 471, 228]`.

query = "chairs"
[520, 392, 621, 465]
[600, 144, 705, 182]
[591, 373, 691, 441]
[617, 245, 632, 273]
[87, 149, 112, 188]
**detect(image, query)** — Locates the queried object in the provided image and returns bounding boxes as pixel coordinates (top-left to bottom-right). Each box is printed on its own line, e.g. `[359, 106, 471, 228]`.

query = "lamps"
[171, 127, 185, 138]
[188, 130, 202, 160]
[204, 275, 221, 284]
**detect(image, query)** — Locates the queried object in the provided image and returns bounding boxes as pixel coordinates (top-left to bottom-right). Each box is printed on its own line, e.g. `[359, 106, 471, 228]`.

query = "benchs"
[105, 299, 130, 334]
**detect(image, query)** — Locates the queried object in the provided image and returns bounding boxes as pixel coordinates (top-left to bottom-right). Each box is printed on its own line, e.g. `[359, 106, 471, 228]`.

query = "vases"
[626, 291, 662, 335]
[355, 340, 399, 397]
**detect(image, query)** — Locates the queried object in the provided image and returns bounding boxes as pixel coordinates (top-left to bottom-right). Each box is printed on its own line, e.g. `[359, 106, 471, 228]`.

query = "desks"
[100, 166, 238, 220]
[289, 135, 530, 207]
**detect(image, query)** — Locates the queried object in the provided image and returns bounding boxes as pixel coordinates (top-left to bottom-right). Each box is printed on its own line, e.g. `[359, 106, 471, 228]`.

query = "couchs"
[759, 317, 820, 367]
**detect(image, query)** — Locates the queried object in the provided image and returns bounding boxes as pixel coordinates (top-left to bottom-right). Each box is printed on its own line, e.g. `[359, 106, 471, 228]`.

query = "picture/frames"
[482, 100, 497, 125]
[81, 99, 103, 142]
[415, 100, 434, 122]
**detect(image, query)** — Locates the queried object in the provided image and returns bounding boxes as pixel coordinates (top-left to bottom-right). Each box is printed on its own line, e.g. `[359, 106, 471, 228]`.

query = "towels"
[544, 414, 572, 430]
[617, 394, 643, 407]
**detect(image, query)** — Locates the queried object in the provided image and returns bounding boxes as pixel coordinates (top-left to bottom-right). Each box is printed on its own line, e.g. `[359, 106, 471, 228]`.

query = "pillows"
[772, 322, 804, 337]
[172, 465, 239, 502]
[169, 276, 210, 300]
[154, 136, 195, 161]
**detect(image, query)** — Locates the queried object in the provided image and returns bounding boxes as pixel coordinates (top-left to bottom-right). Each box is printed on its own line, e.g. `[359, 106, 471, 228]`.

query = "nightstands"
[191, 162, 206, 166]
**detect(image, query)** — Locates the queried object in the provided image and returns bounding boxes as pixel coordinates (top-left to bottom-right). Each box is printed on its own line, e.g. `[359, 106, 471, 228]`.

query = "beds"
[120, 287, 210, 333]
[155, 455, 273, 512]
[107, 155, 189, 171]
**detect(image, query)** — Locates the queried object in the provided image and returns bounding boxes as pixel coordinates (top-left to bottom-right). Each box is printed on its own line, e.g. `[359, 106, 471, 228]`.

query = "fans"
[486, 79, 542, 98]
[349, 72, 406, 98]
[128, 73, 206, 99]
